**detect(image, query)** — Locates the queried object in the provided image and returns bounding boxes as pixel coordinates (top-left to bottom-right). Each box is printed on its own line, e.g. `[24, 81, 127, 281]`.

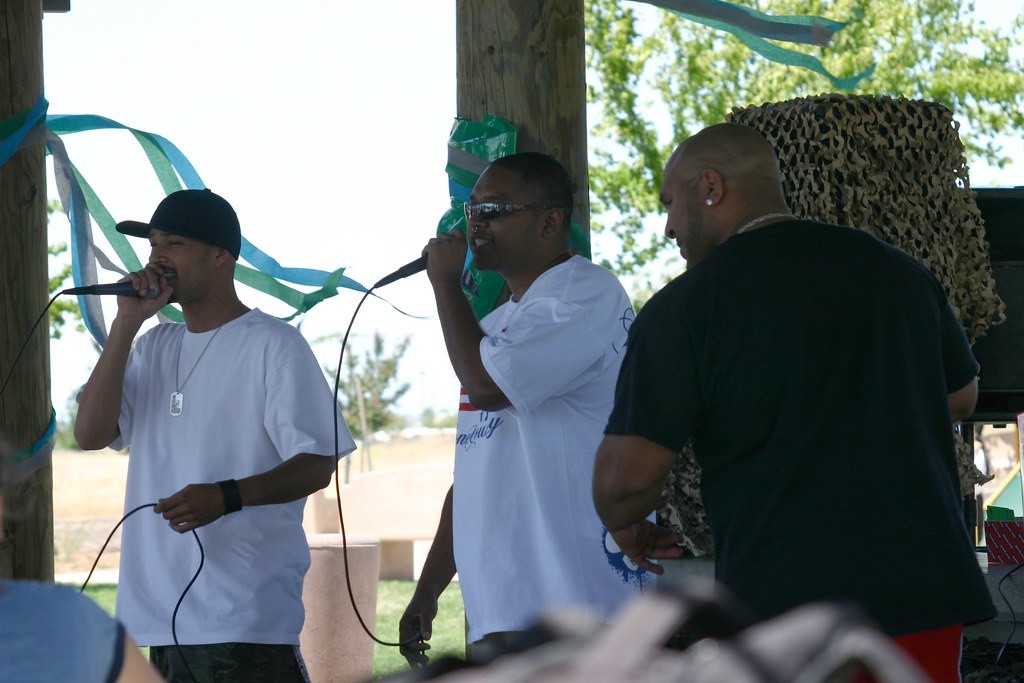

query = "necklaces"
[170, 301, 241, 417]
[738, 213, 796, 234]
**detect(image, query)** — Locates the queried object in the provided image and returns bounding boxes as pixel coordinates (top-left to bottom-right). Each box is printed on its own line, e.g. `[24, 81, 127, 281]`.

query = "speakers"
[951, 187, 1024, 424]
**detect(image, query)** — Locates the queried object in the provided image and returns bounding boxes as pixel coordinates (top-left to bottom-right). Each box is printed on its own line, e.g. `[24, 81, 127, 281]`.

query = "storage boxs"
[984, 520, 1024, 563]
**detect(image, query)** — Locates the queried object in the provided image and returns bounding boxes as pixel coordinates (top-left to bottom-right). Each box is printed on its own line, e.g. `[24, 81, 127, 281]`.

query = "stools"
[380, 535, 435, 580]
[295, 533, 381, 683]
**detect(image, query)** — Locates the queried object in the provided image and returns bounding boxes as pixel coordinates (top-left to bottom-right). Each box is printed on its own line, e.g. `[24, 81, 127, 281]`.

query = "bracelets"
[215, 479, 242, 516]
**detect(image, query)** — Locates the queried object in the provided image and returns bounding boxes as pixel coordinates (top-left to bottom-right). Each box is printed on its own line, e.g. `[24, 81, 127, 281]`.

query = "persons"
[592, 123, 998, 683]
[74, 188, 356, 683]
[0, 580, 166, 683]
[399, 153, 659, 670]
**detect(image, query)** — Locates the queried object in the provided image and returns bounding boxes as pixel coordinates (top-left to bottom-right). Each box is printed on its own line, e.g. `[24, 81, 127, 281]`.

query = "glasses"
[464, 201, 567, 221]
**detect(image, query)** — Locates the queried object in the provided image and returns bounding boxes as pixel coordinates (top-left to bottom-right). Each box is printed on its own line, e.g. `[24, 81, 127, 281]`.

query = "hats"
[115, 188, 241, 260]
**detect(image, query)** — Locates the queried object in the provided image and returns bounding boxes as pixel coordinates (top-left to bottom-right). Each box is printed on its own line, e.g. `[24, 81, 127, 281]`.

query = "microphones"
[373, 254, 428, 289]
[62, 281, 162, 300]
